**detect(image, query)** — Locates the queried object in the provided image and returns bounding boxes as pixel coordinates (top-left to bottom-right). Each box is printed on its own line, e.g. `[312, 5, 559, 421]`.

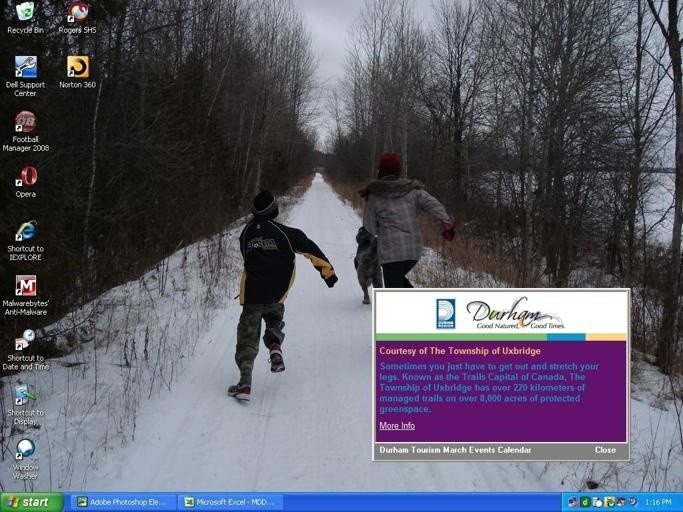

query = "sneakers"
[267, 343, 285, 373]
[228, 383, 251, 401]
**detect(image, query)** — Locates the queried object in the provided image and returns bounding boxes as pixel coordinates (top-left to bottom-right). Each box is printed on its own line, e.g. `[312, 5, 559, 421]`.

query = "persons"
[360, 151, 458, 287]
[224, 188, 339, 403]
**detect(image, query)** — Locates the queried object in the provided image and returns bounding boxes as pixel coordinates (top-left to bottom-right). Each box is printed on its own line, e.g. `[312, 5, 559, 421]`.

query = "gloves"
[325, 274, 338, 288]
[442, 228, 455, 242]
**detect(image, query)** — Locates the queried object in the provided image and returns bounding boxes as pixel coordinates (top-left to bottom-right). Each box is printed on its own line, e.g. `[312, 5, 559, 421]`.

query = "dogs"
[353, 225, 385, 305]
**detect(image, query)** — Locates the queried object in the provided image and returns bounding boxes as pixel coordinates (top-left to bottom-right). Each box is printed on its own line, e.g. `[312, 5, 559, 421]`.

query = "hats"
[251, 190, 278, 220]
[377, 154, 401, 180]
